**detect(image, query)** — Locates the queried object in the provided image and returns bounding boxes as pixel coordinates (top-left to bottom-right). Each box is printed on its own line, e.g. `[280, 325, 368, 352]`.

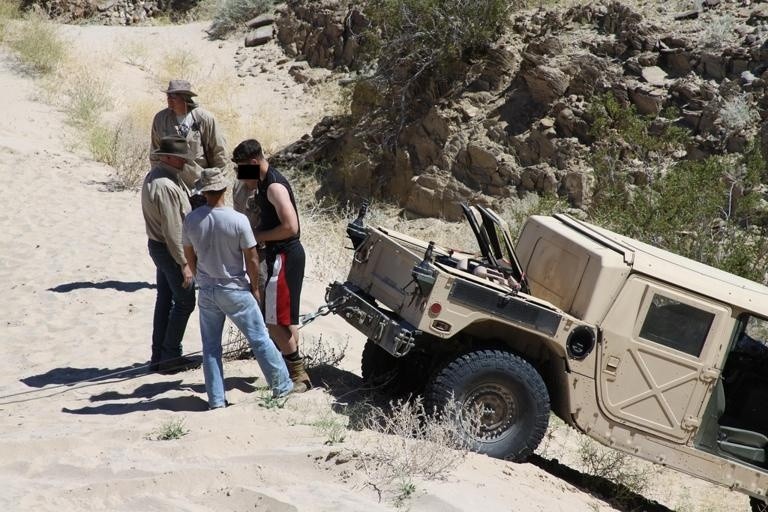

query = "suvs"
[323, 198, 767, 512]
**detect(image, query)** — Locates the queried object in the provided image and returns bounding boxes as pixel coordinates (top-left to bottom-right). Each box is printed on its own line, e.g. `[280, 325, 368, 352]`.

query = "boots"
[285, 359, 310, 387]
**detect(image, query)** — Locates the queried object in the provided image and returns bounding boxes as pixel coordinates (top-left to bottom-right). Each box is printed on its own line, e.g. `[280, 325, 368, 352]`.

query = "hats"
[152, 136, 195, 161]
[161, 80, 199, 96]
[194, 168, 231, 192]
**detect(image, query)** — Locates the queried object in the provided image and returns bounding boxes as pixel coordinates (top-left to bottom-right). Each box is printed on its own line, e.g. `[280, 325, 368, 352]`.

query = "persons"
[232, 165, 269, 311]
[141, 134, 201, 374]
[230, 138, 314, 394]
[149, 80, 232, 209]
[181, 166, 308, 409]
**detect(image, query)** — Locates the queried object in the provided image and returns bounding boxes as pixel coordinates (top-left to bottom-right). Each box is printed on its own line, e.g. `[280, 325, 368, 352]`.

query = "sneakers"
[151, 355, 199, 371]
[241, 348, 254, 359]
[271, 381, 307, 397]
